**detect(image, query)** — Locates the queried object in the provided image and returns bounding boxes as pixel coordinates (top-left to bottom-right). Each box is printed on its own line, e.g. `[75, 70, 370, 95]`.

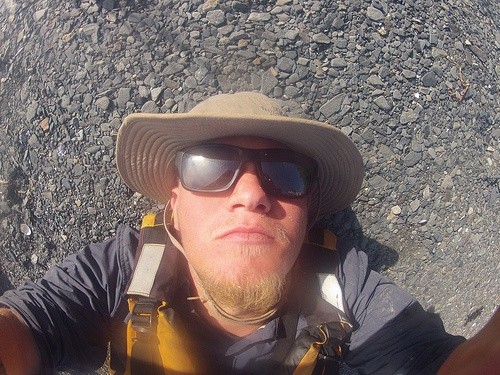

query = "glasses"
[174, 143, 320, 200]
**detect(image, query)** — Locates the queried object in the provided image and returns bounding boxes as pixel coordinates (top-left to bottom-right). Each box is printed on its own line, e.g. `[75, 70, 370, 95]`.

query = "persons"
[0, 91, 500, 375]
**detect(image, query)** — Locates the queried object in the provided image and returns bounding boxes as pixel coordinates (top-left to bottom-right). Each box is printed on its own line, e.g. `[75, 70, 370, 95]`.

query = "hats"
[114, 91, 365, 223]
